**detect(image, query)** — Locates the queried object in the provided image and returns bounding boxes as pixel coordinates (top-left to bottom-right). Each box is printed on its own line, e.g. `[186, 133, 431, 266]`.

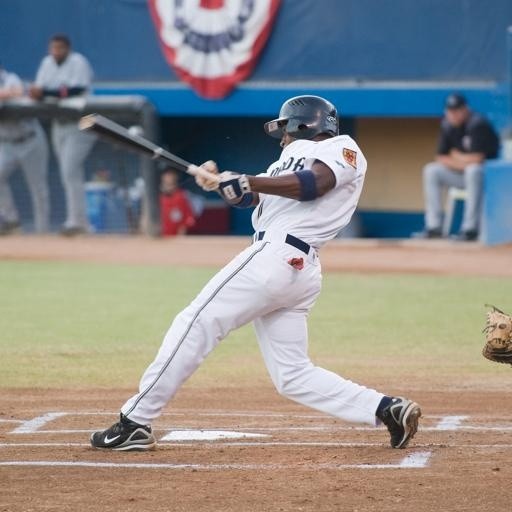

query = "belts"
[256, 231, 310, 255]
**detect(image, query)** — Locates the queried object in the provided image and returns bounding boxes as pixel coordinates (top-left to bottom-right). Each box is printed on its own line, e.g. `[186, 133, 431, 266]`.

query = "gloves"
[194, 160, 252, 206]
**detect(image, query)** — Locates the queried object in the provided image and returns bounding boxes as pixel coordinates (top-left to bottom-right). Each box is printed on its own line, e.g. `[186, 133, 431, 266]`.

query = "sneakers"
[380, 396, 421, 449]
[91, 412, 157, 453]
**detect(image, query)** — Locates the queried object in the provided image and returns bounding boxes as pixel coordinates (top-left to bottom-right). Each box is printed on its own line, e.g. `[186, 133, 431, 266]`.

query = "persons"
[423, 93, 502, 241]
[0, 66, 50, 235]
[89, 95, 422, 454]
[158, 170, 202, 236]
[31, 36, 92, 234]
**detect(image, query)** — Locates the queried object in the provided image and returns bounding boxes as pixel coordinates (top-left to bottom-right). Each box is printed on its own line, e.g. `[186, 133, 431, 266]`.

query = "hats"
[446, 93, 466, 110]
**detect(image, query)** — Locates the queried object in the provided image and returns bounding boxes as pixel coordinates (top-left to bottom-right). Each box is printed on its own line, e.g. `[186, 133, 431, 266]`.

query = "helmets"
[264, 95, 339, 140]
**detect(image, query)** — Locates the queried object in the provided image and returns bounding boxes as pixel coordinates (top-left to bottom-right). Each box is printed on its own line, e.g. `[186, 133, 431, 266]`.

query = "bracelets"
[298, 170, 315, 205]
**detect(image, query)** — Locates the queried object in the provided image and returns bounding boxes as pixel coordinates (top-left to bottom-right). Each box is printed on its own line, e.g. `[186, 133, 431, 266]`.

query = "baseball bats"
[79, 113, 222, 183]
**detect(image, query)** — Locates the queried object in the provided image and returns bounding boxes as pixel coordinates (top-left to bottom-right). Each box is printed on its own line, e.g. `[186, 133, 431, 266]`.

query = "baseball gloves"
[481, 304, 511, 364]
